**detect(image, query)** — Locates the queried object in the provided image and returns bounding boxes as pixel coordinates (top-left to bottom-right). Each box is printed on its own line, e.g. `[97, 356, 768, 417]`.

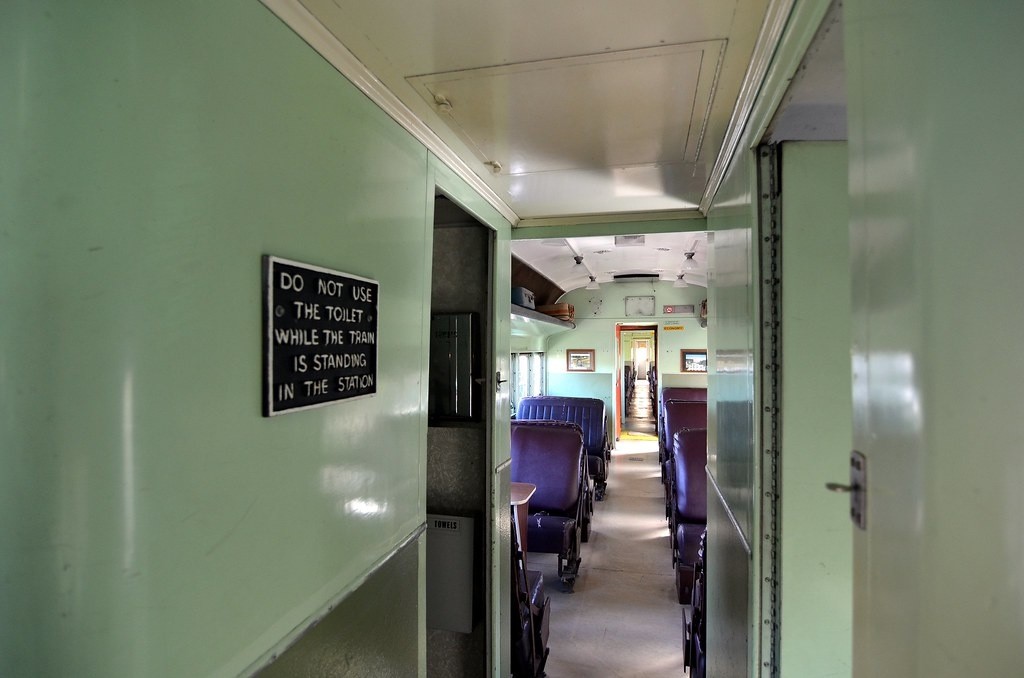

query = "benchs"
[647, 360, 708, 662]
[512, 362, 636, 678]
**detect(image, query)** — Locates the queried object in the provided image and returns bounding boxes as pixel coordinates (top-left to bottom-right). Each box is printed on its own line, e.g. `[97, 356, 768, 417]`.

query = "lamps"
[571, 256, 587, 274]
[672, 274, 690, 288]
[585, 275, 601, 289]
[680, 251, 699, 269]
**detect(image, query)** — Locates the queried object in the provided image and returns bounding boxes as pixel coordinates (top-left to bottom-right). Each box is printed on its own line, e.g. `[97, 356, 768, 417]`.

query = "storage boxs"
[536, 303, 574, 322]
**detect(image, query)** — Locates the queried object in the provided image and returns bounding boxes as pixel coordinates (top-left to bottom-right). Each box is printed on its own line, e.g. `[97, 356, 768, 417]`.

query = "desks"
[510, 481, 537, 569]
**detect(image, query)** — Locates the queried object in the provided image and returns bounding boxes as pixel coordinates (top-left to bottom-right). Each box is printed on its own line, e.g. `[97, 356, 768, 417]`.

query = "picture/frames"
[566, 348, 595, 372]
[680, 349, 708, 373]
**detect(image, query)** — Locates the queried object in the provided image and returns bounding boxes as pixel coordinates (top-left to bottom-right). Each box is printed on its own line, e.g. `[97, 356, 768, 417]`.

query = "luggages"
[511, 286, 535, 310]
[536, 303, 574, 321]
